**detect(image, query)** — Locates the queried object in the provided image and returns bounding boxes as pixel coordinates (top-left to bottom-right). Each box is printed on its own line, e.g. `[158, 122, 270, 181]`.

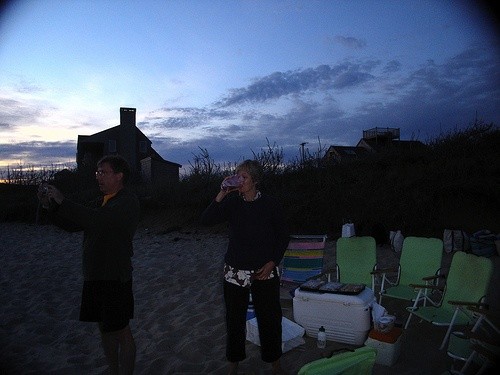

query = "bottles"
[317, 326, 327, 349]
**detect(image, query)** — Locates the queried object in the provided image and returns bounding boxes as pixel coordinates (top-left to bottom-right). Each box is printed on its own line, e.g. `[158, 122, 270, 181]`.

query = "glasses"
[95, 170, 113, 176]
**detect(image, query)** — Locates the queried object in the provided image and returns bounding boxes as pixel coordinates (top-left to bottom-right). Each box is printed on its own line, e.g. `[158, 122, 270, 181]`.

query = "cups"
[220, 174, 244, 192]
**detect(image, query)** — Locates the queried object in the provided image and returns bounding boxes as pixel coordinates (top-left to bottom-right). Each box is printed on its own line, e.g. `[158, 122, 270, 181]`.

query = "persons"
[37, 154, 141, 375]
[199, 161, 290, 375]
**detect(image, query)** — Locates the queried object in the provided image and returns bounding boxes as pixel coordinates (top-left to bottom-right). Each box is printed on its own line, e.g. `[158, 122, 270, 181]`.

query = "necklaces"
[242, 194, 249, 200]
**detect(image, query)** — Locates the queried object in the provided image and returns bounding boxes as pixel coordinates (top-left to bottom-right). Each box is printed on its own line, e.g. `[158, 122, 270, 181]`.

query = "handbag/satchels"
[246, 315, 306, 354]
[470, 229, 497, 257]
[389, 230, 404, 253]
[223, 262, 255, 288]
[442, 225, 470, 254]
[341, 218, 355, 237]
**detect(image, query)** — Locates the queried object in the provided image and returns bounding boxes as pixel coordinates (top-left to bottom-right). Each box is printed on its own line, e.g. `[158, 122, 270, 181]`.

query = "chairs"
[446, 306, 500, 375]
[404, 251, 494, 350]
[323, 236, 383, 296]
[377, 236, 444, 330]
[296, 344, 377, 375]
[279, 234, 327, 300]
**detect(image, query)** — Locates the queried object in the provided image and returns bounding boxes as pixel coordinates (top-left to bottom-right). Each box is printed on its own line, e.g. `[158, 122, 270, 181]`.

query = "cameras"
[41, 186, 49, 193]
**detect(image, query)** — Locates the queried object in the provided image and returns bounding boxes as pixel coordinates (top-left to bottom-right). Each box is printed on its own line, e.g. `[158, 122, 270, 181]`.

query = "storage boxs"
[293, 286, 376, 345]
[246, 305, 257, 320]
[364, 326, 402, 366]
